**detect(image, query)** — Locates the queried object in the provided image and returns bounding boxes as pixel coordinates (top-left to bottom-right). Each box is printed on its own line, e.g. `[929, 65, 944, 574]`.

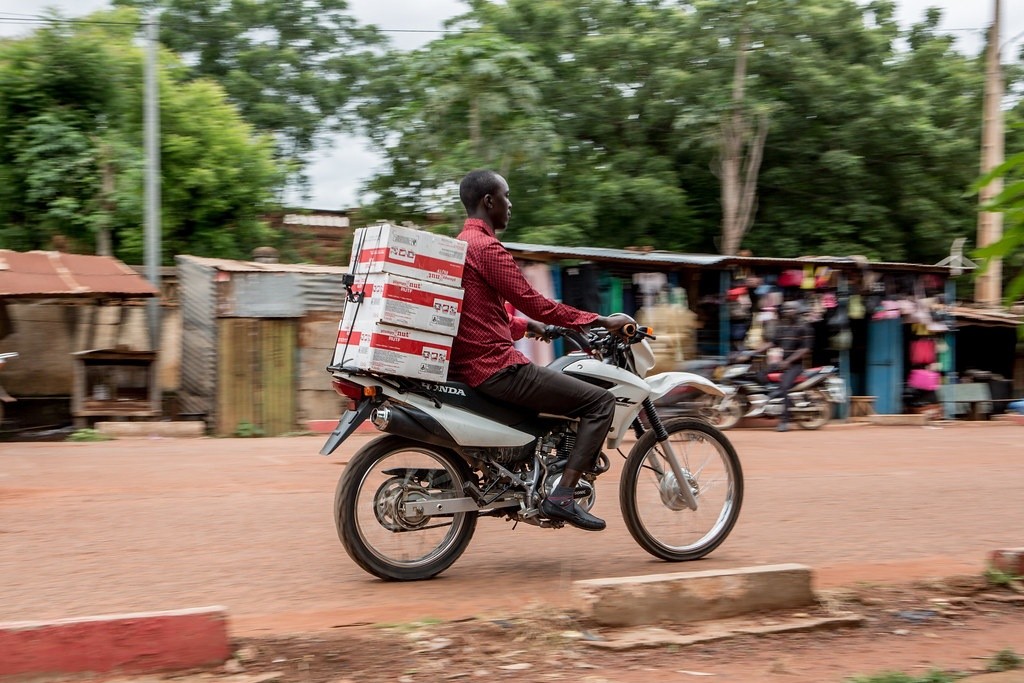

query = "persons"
[741, 299, 807, 431]
[448, 171, 638, 532]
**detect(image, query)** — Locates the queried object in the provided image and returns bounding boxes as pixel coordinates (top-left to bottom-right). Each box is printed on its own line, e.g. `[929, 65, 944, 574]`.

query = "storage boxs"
[347, 224, 469, 288]
[332, 319, 454, 382]
[342, 272, 464, 336]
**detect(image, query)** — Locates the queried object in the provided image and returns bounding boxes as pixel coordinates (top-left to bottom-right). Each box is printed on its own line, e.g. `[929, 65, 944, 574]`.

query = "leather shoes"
[539, 497, 606, 531]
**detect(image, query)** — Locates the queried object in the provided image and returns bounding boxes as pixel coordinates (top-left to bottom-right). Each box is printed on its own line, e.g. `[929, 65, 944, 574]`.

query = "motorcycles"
[318, 312, 744, 583]
[697, 336, 849, 428]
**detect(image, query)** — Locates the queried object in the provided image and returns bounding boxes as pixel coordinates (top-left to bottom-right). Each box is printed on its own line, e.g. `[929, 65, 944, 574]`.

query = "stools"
[848, 395, 880, 416]
